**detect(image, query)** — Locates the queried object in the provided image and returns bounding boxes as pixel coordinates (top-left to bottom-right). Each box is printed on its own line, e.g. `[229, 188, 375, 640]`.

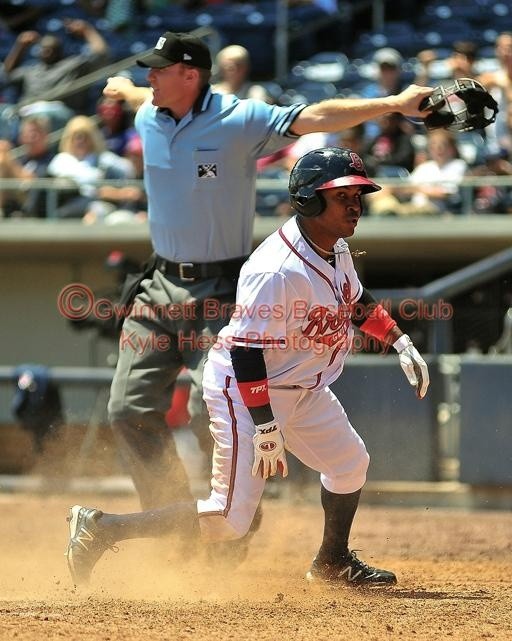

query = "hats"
[136, 32, 212, 69]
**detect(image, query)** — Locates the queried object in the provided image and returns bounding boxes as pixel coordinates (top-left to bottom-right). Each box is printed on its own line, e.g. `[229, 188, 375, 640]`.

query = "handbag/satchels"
[115, 265, 153, 332]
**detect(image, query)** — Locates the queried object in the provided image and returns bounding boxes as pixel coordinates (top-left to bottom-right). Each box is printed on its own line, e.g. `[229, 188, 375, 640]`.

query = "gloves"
[250, 418, 288, 480]
[393, 334, 429, 399]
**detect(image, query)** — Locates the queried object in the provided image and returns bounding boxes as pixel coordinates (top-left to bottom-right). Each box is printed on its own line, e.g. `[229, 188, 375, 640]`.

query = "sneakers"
[306, 548, 397, 589]
[206, 501, 263, 571]
[66, 504, 116, 591]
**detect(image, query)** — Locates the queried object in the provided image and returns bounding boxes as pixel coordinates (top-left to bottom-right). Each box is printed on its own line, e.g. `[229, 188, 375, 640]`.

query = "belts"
[152, 254, 250, 281]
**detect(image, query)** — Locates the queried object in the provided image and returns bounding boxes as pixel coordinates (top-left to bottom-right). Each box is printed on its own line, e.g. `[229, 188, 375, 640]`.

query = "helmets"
[289, 146, 382, 217]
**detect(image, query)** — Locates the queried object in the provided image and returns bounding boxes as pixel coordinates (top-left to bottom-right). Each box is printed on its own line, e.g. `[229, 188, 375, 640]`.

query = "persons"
[97, 31, 439, 582]
[68, 144, 432, 590]
[2, 2, 512, 220]
